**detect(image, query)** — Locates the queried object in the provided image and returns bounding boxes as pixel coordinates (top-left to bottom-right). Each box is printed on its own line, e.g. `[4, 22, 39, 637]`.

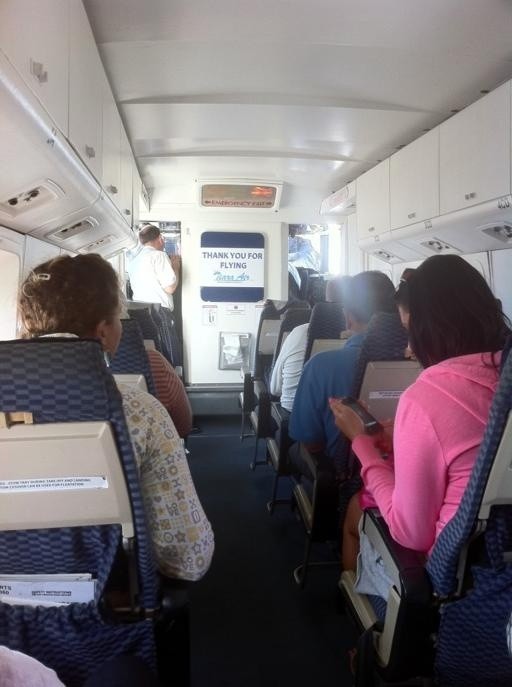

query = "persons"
[127, 224, 203, 435]
[268, 273, 351, 452]
[326, 254, 511, 679]
[283, 270, 396, 587]
[16, 252, 216, 584]
[145, 348, 193, 436]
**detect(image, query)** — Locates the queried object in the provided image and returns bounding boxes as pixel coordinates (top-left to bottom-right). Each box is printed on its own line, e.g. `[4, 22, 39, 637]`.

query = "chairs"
[0, 298, 186, 681]
[238, 299, 512, 682]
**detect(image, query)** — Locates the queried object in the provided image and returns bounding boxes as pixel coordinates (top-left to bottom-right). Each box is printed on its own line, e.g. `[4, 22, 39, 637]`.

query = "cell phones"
[339, 396, 384, 436]
